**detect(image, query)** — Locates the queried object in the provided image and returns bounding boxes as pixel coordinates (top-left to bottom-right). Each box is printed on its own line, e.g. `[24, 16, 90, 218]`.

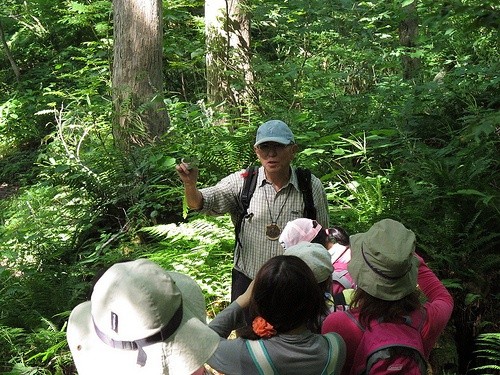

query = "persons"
[205, 254, 346, 375]
[278, 218, 360, 296]
[281, 241, 358, 333]
[175, 119, 331, 340]
[320, 219, 454, 375]
[66, 259, 222, 375]
[173, 120, 332, 339]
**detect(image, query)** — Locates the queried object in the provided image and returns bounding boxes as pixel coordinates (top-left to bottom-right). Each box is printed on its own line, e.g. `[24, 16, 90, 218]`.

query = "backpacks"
[345, 307, 429, 375]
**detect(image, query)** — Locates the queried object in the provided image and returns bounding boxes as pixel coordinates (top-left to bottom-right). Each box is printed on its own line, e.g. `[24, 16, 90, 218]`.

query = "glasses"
[256, 143, 291, 151]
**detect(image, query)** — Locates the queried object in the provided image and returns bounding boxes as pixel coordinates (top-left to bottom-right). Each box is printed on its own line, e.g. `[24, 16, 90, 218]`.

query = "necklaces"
[262, 180, 293, 241]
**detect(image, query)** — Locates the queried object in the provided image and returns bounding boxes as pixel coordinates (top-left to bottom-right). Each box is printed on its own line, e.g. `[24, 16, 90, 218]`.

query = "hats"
[254, 120, 295, 148]
[67, 258, 220, 374]
[283, 241, 334, 283]
[278, 217, 322, 252]
[348, 219, 420, 300]
